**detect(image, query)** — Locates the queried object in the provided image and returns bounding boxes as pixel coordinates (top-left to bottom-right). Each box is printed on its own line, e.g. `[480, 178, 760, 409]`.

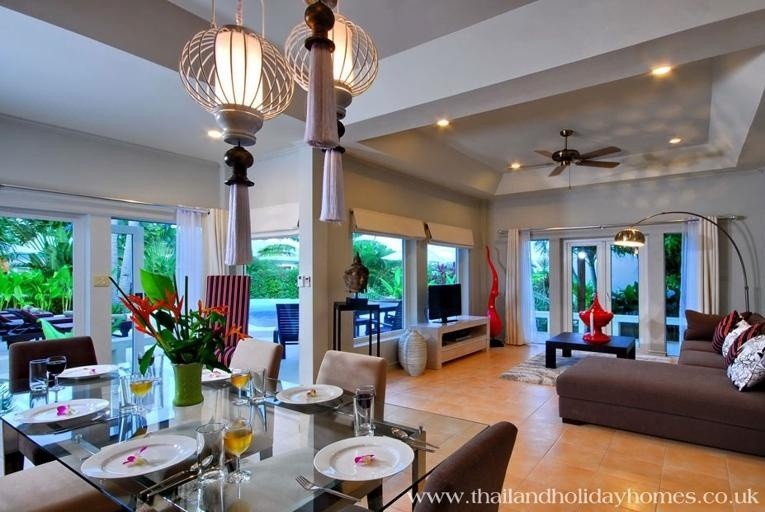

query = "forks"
[293, 473, 360, 503]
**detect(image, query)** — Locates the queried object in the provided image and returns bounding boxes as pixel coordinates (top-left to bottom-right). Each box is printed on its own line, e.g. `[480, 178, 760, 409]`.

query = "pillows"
[711, 310, 765, 392]
[684, 310, 721, 341]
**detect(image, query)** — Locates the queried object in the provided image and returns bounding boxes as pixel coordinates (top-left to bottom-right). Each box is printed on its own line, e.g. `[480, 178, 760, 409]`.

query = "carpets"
[499, 349, 679, 388]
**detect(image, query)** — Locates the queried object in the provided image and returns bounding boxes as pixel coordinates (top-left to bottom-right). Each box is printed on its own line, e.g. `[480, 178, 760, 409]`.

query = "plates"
[200, 365, 234, 383]
[81, 435, 197, 479]
[313, 435, 414, 480]
[275, 383, 343, 404]
[12, 397, 109, 424]
[56, 364, 119, 380]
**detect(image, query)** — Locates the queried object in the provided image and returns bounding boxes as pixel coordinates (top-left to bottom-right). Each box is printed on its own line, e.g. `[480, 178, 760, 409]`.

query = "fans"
[520, 129, 621, 177]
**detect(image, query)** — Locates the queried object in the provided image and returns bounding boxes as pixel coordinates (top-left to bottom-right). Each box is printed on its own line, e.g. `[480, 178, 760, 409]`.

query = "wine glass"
[357, 384, 376, 429]
[130, 372, 154, 416]
[230, 369, 249, 404]
[46, 356, 66, 391]
[233, 407, 251, 432]
[220, 416, 252, 483]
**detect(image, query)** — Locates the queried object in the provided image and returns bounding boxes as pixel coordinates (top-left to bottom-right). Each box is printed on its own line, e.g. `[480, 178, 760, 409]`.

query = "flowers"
[106, 275, 253, 376]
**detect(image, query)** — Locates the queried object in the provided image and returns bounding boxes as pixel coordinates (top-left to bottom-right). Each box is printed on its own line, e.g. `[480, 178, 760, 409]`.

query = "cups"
[249, 405, 266, 432]
[118, 414, 147, 441]
[196, 423, 224, 481]
[0, 378, 14, 411]
[250, 368, 267, 404]
[137, 353, 153, 378]
[28, 390, 49, 409]
[352, 393, 376, 436]
[28, 359, 49, 391]
[118, 375, 137, 413]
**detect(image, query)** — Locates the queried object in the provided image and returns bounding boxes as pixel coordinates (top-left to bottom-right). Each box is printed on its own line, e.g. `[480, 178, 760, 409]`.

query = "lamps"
[614, 212, 749, 313]
[177, 1, 294, 266]
[578, 246, 587, 260]
[284, 0, 378, 226]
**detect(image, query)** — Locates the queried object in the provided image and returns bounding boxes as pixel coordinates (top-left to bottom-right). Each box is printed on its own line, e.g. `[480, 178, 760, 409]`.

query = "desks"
[409, 316, 490, 370]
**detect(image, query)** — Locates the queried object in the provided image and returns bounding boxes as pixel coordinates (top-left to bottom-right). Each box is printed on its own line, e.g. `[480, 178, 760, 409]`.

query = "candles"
[590, 311, 594, 337]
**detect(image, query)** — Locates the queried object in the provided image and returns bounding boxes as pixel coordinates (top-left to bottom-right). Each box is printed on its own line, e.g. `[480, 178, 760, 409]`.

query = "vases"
[398, 328, 428, 377]
[172, 361, 204, 407]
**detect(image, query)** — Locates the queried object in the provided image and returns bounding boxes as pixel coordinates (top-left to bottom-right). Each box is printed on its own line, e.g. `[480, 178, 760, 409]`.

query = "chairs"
[274, 305, 300, 359]
[353, 299, 402, 338]
[0, 305, 133, 393]
[316, 350, 386, 511]
[202, 336, 282, 461]
[0, 452, 135, 512]
[345, 421, 517, 512]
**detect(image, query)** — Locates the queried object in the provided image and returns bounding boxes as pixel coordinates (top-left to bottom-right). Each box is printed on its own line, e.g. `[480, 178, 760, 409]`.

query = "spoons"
[49, 410, 109, 433]
[390, 427, 440, 449]
[140, 452, 212, 495]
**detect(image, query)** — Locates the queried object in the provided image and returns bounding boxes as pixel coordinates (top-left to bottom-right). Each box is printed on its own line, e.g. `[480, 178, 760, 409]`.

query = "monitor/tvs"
[428, 284, 462, 323]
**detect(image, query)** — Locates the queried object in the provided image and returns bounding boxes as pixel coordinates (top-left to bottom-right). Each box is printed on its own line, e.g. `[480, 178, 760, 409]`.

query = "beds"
[555, 354, 765, 457]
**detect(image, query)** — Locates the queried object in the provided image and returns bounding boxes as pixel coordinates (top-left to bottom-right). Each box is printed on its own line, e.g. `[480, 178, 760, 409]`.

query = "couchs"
[678, 310, 765, 368]
